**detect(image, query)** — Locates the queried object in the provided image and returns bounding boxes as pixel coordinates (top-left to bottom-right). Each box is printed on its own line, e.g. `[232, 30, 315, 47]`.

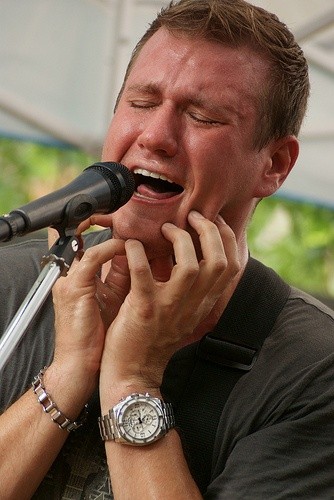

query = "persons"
[0, 0, 334, 500]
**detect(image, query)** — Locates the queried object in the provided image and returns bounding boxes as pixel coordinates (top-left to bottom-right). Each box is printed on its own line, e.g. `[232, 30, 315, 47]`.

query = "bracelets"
[32, 366, 88, 434]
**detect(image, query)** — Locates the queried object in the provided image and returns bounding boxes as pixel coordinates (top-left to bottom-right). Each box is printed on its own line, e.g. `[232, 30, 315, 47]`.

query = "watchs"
[97, 393, 176, 447]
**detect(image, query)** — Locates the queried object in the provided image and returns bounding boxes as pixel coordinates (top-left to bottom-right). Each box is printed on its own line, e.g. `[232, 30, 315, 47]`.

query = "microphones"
[0, 162, 137, 245]
